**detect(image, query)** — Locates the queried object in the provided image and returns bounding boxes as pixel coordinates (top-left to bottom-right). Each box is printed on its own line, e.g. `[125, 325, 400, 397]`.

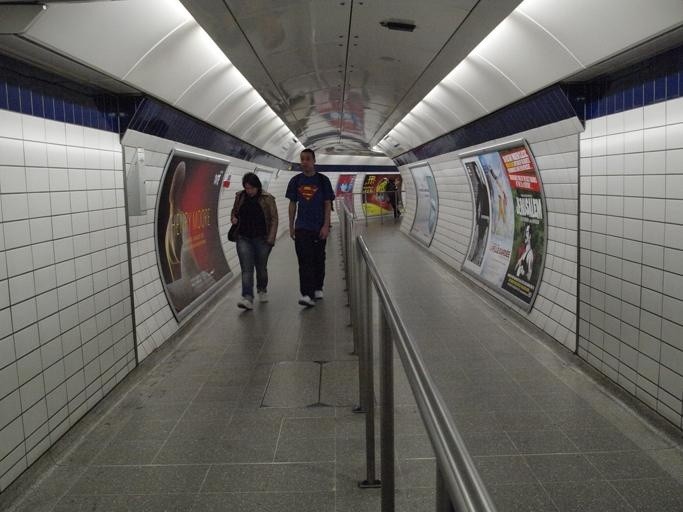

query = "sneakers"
[298, 290, 323, 306]
[258, 292, 269, 303]
[236, 294, 254, 310]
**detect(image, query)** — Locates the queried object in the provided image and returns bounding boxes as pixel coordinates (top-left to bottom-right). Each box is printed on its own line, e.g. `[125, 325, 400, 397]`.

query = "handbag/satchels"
[228, 223, 242, 242]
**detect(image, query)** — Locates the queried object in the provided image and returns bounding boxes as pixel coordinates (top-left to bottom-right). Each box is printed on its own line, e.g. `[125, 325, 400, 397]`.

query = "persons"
[226, 172, 278, 310]
[284, 148, 335, 308]
[385, 178, 400, 218]
[512, 222, 534, 280]
